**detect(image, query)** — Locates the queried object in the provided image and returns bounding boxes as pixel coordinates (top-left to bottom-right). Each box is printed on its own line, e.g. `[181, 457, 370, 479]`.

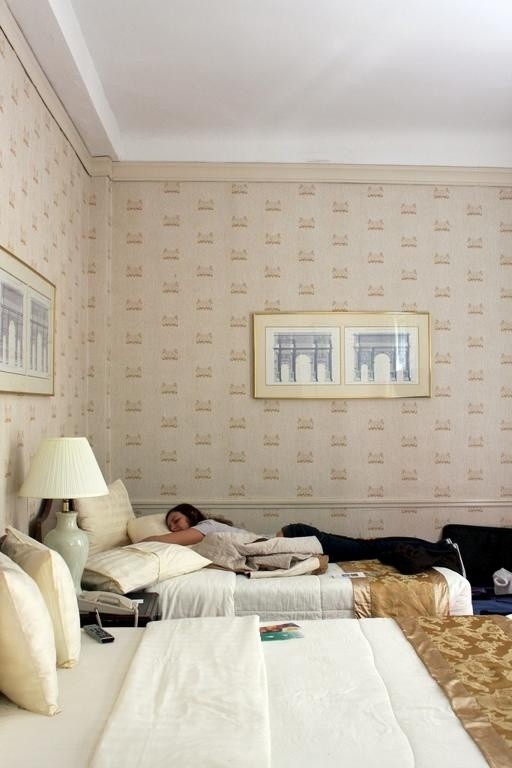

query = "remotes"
[84, 623, 115, 644]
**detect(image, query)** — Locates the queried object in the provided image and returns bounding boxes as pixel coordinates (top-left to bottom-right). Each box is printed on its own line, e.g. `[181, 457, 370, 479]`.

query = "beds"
[28, 484, 474, 627]
[1, 543, 510, 766]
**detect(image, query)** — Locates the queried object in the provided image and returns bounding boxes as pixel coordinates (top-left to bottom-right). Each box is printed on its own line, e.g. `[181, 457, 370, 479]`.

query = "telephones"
[77, 589, 139, 616]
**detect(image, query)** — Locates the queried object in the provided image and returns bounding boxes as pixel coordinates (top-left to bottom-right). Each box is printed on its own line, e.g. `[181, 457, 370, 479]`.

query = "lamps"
[19, 437, 112, 599]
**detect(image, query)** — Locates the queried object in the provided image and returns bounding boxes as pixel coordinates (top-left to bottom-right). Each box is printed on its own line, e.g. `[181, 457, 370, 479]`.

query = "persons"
[134, 502, 469, 584]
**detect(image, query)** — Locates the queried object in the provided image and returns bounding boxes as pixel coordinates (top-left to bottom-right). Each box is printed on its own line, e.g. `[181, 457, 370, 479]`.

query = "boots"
[426, 537, 467, 579]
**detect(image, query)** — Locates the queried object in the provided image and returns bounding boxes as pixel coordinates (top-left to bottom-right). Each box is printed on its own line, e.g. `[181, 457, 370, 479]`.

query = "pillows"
[5, 525, 82, 671]
[74, 481, 212, 594]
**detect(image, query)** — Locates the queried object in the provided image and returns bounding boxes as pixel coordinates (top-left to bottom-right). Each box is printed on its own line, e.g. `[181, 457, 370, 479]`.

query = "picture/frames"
[0, 244, 63, 398]
[253, 306, 433, 400]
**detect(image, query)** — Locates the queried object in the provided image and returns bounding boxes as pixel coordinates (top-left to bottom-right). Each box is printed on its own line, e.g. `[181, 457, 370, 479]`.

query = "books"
[260, 621, 304, 635]
[260, 632, 305, 641]
